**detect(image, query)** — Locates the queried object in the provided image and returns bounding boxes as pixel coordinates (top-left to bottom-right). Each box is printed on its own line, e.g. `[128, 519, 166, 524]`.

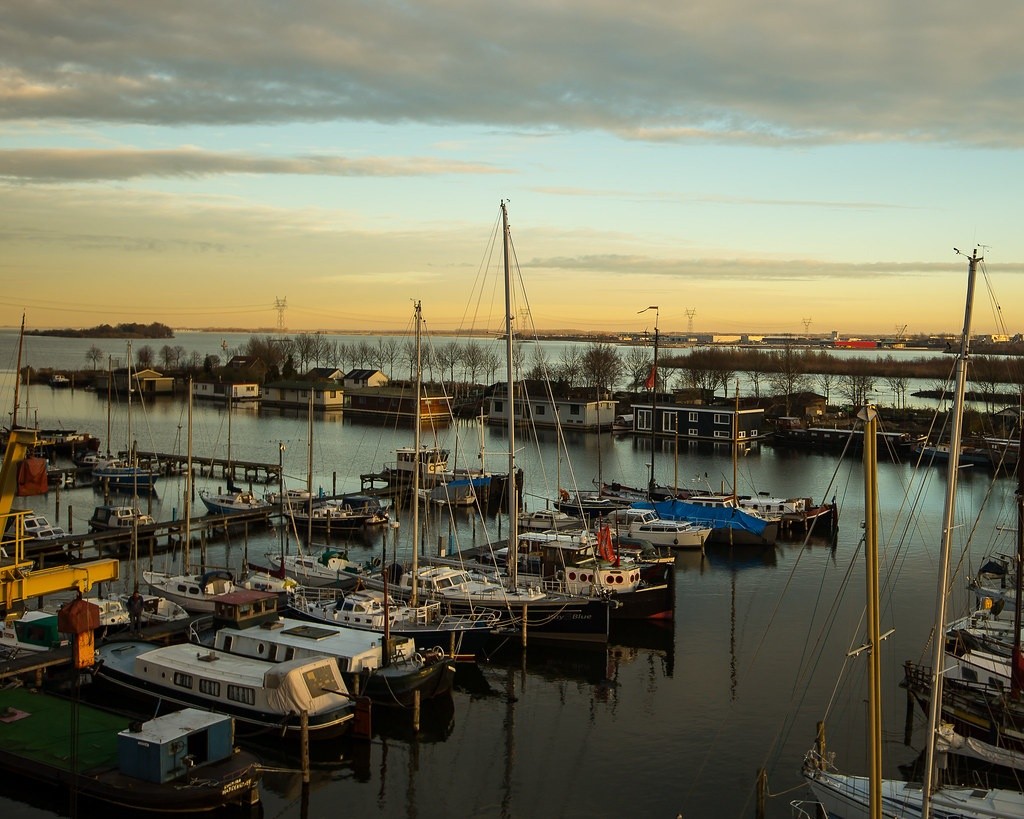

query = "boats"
[1, 547, 377, 670]
[277, 586, 504, 677]
[593, 477, 839, 543]
[364, 560, 614, 648]
[88, 639, 372, 744]
[577, 507, 714, 554]
[468, 530, 678, 622]
[5, 456, 318, 540]
[964, 422, 1022, 609]
[899, 657, 1023, 755]
[911, 435, 1023, 466]
[927, 608, 1024, 698]
[554, 499, 779, 556]
[1, 680, 267, 818]
[799, 398, 1024, 819]
[382, 443, 525, 508]
[187, 588, 458, 713]
[286, 499, 391, 533]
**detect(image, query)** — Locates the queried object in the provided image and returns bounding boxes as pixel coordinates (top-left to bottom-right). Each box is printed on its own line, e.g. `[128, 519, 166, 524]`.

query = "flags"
[596, 524, 616, 563]
